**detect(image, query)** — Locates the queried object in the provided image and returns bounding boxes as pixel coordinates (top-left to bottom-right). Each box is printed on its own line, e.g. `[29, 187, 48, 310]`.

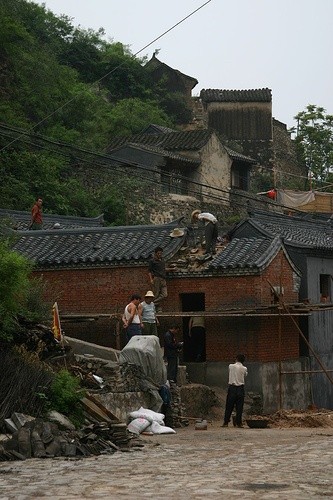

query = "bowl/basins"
[245, 419, 269, 428]
[195, 422, 207, 430]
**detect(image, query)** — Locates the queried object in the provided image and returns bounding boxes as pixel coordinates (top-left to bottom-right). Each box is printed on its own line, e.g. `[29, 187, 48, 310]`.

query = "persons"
[31, 196, 44, 230]
[121, 294, 141, 344]
[160, 324, 184, 386]
[189, 317, 206, 363]
[220, 354, 248, 429]
[191, 210, 219, 255]
[147, 247, 168, 313]
[138, 291, 158, 335]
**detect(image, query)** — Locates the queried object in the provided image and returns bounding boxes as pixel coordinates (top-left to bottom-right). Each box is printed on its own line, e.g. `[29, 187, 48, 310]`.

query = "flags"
[54, 303, 63, 343]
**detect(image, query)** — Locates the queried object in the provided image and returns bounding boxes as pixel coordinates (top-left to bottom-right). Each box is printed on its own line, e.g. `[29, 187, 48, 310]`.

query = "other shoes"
[221, 421, 228, 427]
[236, 424, 243, 428]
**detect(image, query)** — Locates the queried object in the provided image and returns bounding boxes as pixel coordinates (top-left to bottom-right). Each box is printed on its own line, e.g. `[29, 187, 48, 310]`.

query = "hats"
[145, 291, 155, 297]
[170, 228, 184, 237]
[192, 209, 201, 217]
